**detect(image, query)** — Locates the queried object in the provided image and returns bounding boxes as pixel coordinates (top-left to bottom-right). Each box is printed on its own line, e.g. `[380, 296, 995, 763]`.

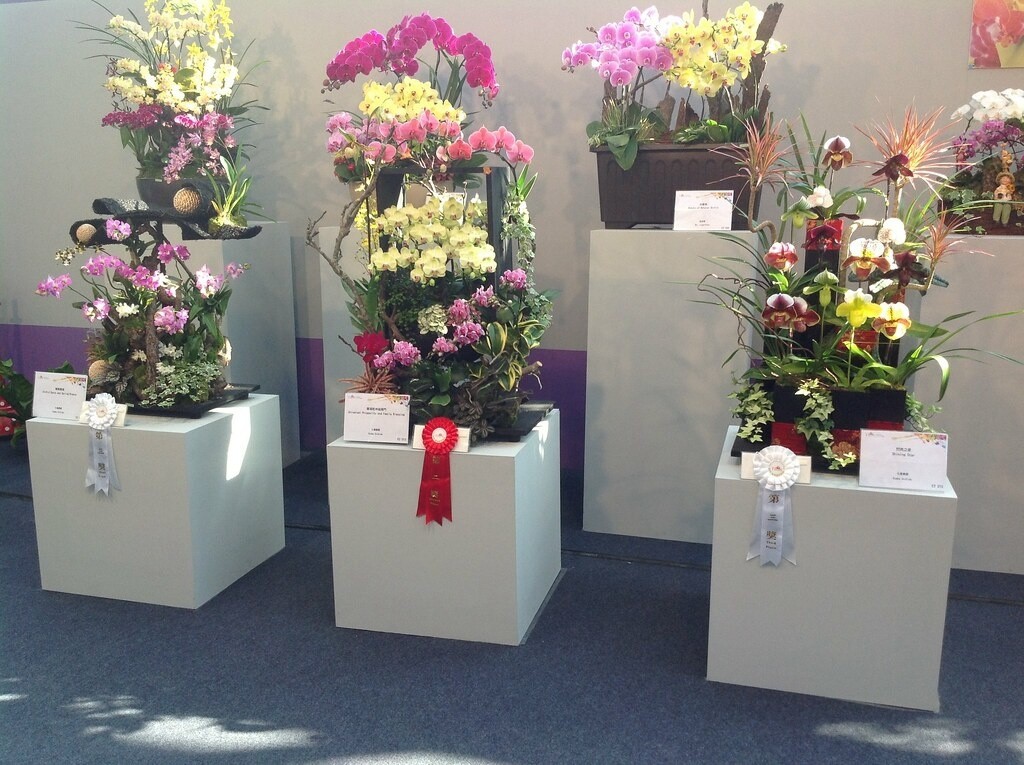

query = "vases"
[770, 381, 907, 475]
[937, 200, 1024, 235]
[589, 142, 763, 230]
[136, 176, 230, 218]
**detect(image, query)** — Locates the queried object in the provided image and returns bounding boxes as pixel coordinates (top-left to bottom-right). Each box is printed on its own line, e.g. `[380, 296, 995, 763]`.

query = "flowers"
[559, 1, 1024, 474]
[33, 218, 251, 408]
[305, 11, 554, 438]
[63, 0, 271, 185]
[949, 87, 1024, 237]
